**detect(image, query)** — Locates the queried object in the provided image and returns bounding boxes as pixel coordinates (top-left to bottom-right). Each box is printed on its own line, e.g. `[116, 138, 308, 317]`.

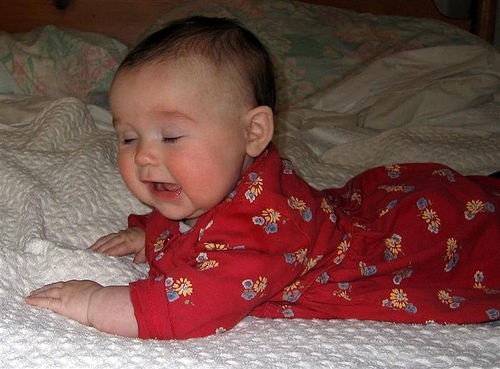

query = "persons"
[26, 16, 500, 343]
